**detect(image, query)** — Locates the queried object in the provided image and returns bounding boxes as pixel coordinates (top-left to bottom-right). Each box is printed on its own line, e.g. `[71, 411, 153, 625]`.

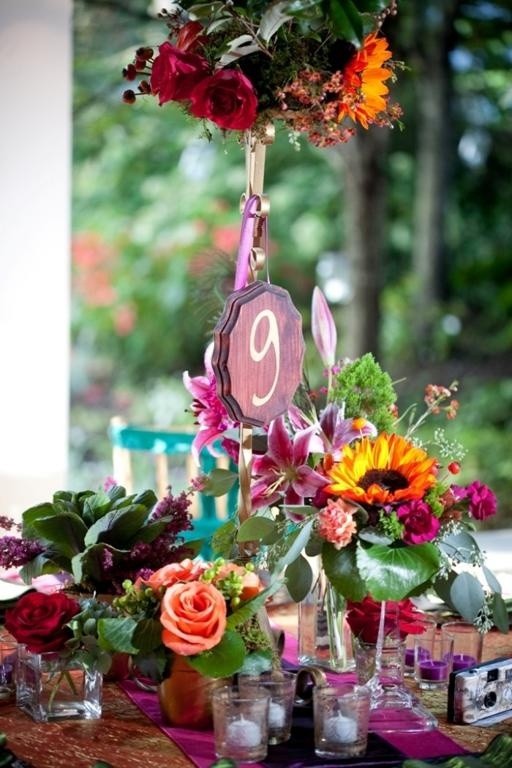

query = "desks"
[0, 611, 512, 767]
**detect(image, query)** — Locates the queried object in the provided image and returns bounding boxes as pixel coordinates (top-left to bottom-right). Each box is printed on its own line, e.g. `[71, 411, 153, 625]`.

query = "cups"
[367, 621, 482, 692]
[311, 683, 371, 759]
[208, 685, 271, 765]
[238, 668, 297, 744]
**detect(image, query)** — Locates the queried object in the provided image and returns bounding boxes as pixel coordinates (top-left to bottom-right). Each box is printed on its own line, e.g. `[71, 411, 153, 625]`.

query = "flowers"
[0, 1, 512, 717]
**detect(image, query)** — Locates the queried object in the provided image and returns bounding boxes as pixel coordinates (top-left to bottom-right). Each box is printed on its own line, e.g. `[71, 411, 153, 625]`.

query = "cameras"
[446, 656, 512, 725]
